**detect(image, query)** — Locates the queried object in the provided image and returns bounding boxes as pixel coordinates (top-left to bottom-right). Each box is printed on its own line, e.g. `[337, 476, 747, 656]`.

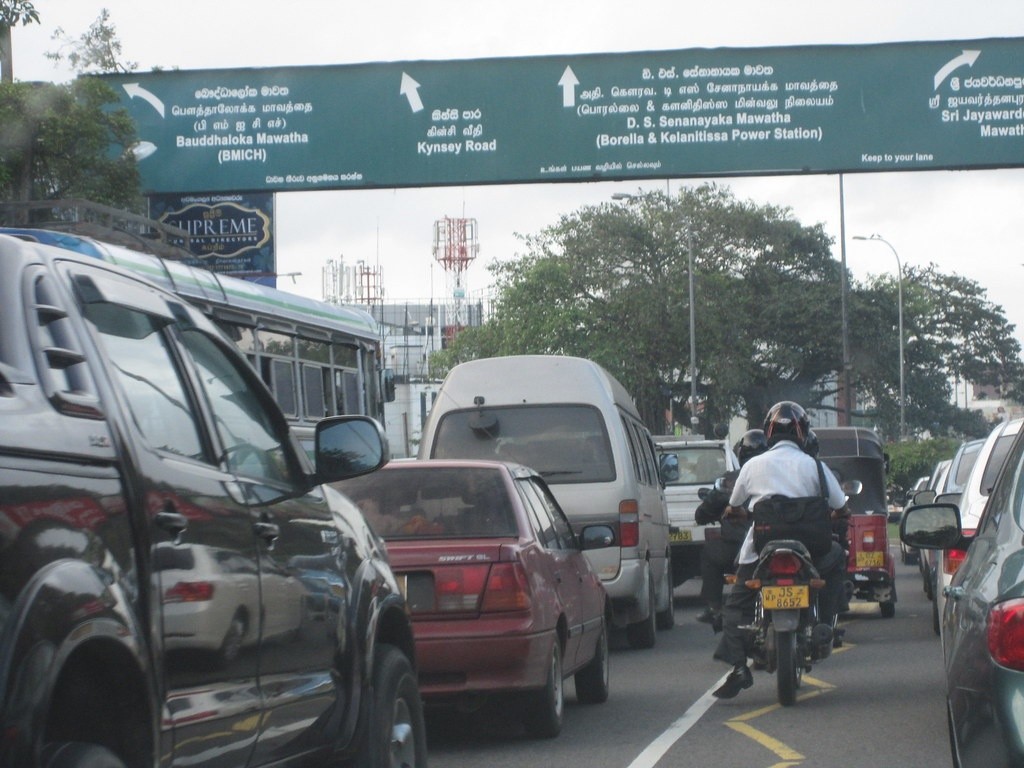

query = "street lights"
[612, 192, 697, 436]
[853, 234, 905, 443]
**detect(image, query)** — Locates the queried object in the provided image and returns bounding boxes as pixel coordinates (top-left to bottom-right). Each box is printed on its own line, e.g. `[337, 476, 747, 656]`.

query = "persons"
[665, 463, 677, 479]
[695, 454, 718, 481]
[712, 401, 852, 699]
[695, 429, 767, 622]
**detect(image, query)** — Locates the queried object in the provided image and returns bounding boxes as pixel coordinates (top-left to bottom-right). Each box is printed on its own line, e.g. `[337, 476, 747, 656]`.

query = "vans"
[419, 354, 680, 649]
[809, 428, 894, 618]
[0, 230, 430, 768]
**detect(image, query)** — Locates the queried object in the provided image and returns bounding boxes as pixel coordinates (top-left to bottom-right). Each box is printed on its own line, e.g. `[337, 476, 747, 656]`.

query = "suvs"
[656, 439, 742, 587]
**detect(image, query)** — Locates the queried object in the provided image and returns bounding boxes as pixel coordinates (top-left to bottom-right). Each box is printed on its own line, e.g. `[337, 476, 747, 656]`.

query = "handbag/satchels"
[752, 493, 832, 560]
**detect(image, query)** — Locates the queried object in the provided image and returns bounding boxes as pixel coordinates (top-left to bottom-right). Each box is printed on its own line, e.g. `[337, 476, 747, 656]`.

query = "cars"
[897, 413, 1024, 768]
[324, 458, 609, 738]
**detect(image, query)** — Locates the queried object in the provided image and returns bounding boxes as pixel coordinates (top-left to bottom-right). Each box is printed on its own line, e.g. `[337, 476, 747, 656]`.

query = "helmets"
[732, 400, 820, 466]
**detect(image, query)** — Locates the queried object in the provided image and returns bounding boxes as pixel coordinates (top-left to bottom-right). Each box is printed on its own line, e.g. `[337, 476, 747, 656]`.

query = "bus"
[0, 226, 394, 470]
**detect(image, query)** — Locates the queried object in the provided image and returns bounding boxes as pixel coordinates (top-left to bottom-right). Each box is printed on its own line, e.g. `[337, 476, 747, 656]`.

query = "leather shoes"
[712, 666, 753, 698]
[696, 607, 723, 622]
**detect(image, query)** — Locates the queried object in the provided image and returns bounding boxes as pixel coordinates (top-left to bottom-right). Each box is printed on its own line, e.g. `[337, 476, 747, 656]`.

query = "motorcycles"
[699, 470, 862, 706]
